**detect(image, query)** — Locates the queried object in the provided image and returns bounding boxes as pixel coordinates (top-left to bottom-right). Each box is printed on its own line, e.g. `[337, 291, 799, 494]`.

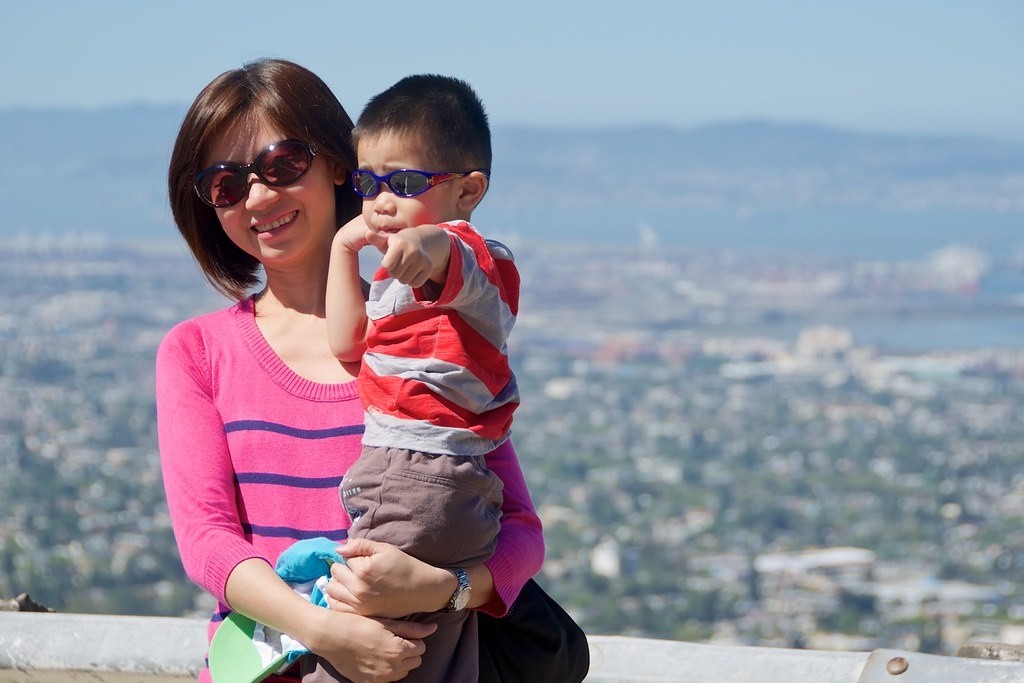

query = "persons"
[155, 57, 546, 683]
[300, 73, 521, 683]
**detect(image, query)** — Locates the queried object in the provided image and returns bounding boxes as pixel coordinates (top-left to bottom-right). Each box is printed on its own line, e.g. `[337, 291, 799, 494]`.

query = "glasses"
[350, 168, 491, 198]
[192, 137, 320, 208]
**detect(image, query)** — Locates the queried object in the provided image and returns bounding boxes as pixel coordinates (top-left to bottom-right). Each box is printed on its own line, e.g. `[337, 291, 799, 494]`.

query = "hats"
[207, 537, 349, 682]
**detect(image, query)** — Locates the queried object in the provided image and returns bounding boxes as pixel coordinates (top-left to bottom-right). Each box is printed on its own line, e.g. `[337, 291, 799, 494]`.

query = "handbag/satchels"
[479, 573, 590, 683]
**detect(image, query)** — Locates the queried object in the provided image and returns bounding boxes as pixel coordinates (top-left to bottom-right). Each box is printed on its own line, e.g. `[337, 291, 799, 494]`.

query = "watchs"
[433, 567, 472, 616]
